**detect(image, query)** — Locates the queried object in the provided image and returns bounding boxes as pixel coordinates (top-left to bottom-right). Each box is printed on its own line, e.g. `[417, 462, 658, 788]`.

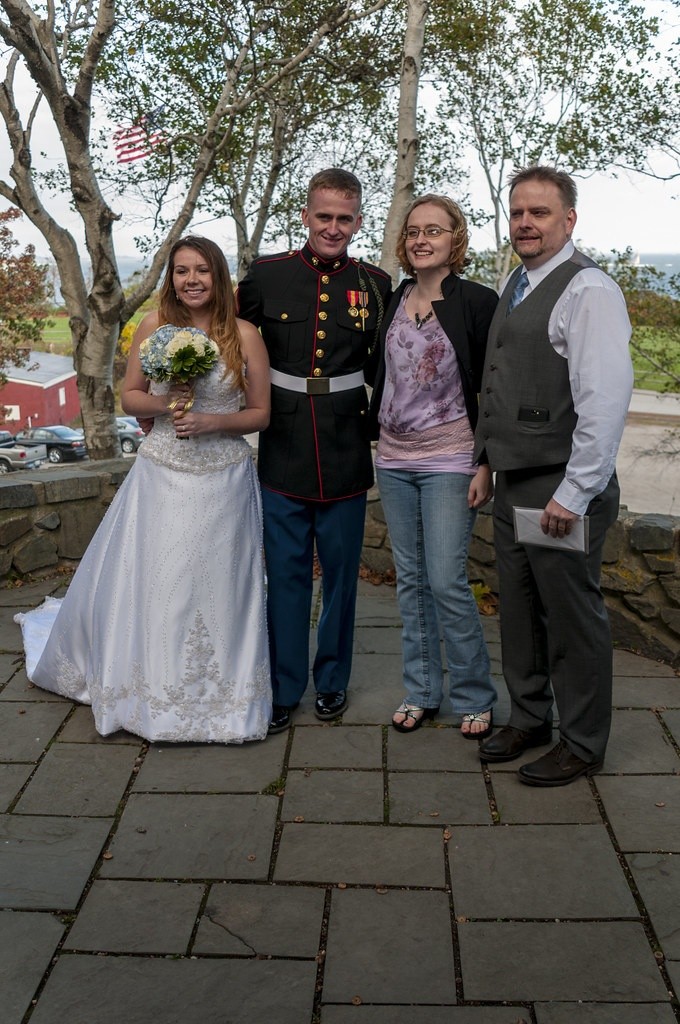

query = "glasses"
[401, 225, 454, 239]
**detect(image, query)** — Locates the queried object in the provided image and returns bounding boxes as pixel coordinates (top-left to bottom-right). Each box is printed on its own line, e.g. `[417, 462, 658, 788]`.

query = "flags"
[114, 105, 167, 163]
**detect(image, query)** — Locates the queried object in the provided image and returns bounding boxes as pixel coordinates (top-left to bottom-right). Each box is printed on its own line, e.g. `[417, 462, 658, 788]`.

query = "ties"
[506, 272, 529, 318]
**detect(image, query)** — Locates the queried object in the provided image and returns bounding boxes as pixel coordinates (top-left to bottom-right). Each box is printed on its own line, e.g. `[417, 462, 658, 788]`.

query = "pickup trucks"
[0, 430, 49, 476]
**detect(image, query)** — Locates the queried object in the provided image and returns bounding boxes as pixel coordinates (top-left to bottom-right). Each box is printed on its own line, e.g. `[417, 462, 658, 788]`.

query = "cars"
[115, 416, 146, 454]
[13, 425, 88, 464]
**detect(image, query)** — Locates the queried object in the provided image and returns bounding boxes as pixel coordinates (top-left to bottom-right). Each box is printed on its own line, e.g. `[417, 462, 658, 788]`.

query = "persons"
[14, 236, 269, 746]
[369, 194, 499, 739]
[237, 169, 392, 731]
[476, 167, 634, 786]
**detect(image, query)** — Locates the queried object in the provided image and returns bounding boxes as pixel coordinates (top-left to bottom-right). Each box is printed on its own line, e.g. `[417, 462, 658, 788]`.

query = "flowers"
[140, 326, 222, 437]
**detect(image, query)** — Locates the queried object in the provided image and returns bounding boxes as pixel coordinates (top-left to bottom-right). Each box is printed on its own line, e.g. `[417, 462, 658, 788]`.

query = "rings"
[183, 426, 185, 431]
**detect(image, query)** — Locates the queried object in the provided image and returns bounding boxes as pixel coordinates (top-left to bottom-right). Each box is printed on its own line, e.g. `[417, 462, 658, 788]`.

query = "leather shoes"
[478, 726, 553, 762]
[517, 743, 602, 786]
[268, 701, 300, 732]
[314, 690, 347, 719]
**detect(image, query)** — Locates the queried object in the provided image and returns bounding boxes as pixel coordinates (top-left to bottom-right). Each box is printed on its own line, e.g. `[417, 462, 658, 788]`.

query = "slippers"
[460, 706, 493, 740]
[391, 701, 439, 732]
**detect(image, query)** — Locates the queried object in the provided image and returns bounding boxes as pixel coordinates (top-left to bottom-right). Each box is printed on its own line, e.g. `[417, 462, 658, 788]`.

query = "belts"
[269, 367, 365, 394]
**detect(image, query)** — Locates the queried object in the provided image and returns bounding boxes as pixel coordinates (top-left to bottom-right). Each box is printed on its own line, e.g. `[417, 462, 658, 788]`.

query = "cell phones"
[517, 404, 550, 422]
